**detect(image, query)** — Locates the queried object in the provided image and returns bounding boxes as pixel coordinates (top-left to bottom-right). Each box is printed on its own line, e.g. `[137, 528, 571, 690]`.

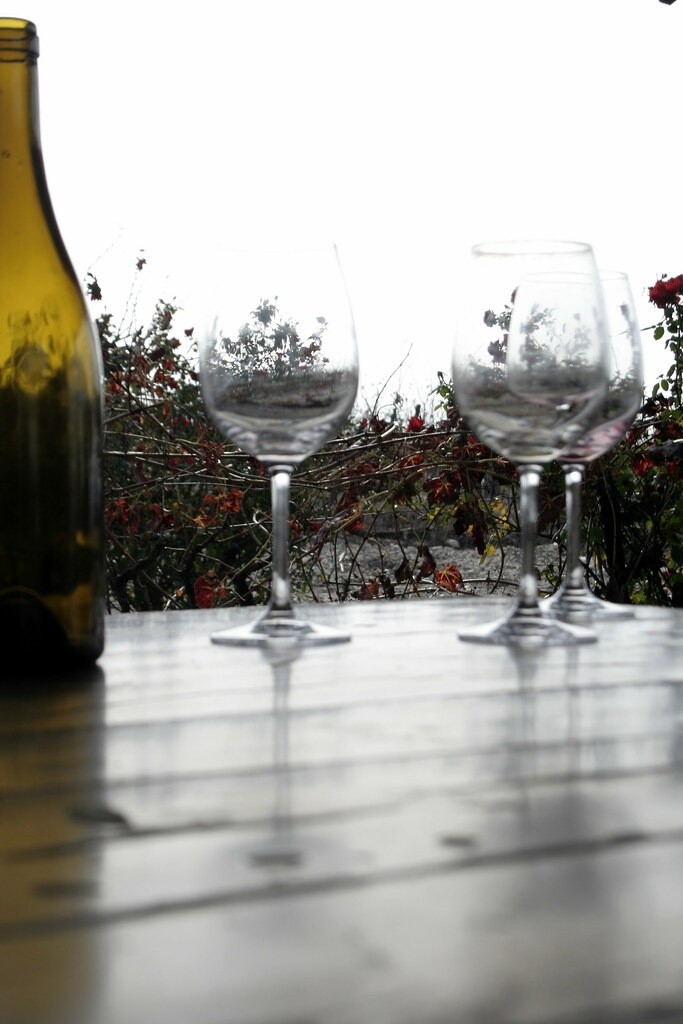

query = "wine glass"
[196, 242, 360, 646]
[506, 270, 644, 623]
[451, 239, 609, 646]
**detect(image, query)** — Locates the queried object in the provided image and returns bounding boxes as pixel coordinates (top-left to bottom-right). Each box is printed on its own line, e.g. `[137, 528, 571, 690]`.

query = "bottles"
[0, 17, 106, 676]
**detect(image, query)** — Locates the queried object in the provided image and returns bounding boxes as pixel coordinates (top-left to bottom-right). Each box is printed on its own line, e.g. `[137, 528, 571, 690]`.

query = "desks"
[0, 598, 683, 1024]
